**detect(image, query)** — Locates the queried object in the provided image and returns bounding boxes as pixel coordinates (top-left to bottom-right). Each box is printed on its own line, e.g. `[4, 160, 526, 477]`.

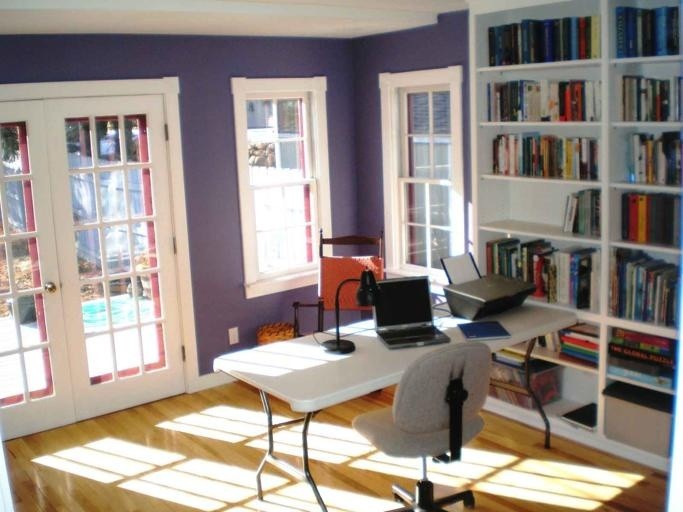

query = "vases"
[127, 281, 144, 298]
[7, 289, 37, 321]
[134, 265, 152, 299]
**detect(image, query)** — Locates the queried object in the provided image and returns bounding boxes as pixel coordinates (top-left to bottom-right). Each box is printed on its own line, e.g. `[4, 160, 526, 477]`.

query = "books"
[493, 131, 682, 186]
[488, 349, 597, 431]
[560, 189, 683, 246]
[456, 320, 509, 340]
[487, 238, 681, 327]
[487, 74, 682, 123]
[488, 7, 680, 66]
[538, 323, 675, 390]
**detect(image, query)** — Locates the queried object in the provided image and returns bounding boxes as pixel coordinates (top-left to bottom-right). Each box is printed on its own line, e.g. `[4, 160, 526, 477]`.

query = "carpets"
[80, 292, 153, 326]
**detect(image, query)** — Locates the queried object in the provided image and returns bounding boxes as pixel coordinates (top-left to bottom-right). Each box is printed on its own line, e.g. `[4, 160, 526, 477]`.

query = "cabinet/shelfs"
[466, 1, 683, 476]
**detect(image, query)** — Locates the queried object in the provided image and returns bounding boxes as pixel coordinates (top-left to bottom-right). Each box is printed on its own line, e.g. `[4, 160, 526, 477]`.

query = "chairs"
[292, 228, 384, 338]
[352, 342, 491, 512]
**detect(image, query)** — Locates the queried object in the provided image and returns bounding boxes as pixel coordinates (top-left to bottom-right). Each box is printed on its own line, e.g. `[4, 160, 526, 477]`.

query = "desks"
[212, 303, 578, 512]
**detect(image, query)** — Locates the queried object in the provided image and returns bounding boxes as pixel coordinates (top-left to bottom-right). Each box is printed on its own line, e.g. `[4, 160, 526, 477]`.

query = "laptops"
[371, 275, 450, 349]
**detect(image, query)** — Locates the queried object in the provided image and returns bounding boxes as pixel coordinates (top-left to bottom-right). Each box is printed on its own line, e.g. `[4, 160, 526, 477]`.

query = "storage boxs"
[490, 362, 558, 407]
[601, 381, 673, 458]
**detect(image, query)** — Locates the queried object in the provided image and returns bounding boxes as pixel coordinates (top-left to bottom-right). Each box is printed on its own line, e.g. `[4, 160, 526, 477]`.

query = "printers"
[440, 252, 536, 320]
